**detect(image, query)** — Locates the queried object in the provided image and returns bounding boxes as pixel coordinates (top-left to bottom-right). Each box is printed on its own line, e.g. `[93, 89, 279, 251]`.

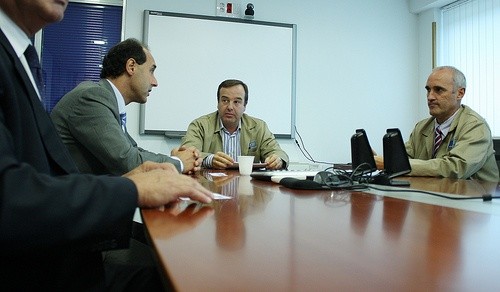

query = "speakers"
[351, 128, 377, 182]
[373, 128, 412, 186]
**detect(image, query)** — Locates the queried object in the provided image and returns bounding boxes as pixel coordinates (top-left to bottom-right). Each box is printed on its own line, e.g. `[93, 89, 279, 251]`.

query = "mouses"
[314, 170, 341, 185]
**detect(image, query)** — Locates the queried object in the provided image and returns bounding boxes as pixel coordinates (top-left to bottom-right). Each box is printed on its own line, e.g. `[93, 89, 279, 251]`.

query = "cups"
[237, 156, 255, 175]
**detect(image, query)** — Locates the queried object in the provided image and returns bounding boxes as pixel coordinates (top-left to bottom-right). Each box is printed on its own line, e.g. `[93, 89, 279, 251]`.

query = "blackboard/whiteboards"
[138, 8, 297, 140]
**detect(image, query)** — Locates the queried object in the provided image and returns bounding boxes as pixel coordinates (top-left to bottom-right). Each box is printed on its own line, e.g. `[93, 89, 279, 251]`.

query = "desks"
[138, 161, 500, 292]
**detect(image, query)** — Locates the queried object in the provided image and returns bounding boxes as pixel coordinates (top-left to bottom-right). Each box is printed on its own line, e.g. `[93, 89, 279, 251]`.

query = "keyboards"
[250, 171, 325, 183]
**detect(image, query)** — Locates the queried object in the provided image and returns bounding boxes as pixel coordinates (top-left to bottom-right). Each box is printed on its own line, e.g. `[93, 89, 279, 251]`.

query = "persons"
[49, 37, 203, 292]
[179, 79, 289, 170]
[373, 66, 500, 182]
[0, 0, 214, 292]
